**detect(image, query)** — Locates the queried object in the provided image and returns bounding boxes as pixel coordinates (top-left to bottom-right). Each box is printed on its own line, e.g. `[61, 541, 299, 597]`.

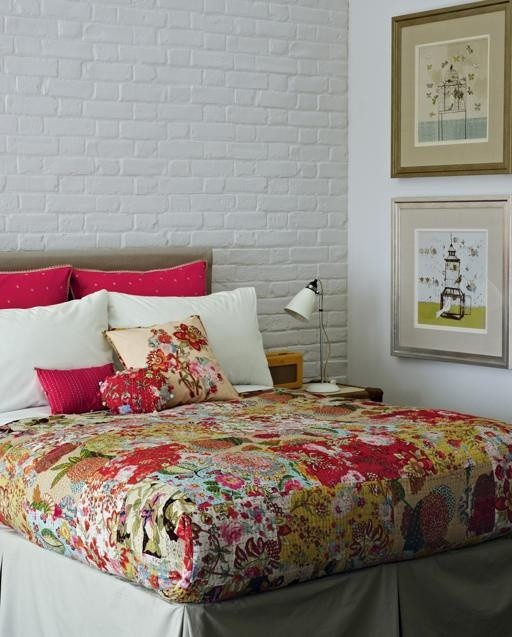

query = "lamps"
[285, 277, 340, 391]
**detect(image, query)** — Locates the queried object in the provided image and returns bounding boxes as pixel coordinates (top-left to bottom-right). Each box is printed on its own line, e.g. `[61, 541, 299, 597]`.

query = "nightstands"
[304, 378, 383, 403]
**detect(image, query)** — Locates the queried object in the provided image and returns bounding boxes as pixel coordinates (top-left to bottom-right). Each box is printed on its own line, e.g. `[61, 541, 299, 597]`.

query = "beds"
[0, 242, 509, 637]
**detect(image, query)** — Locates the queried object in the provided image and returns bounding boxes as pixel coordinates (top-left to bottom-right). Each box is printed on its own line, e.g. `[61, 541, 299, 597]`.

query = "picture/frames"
[389, 0, 511, 175]
[391, 194, 509, 368]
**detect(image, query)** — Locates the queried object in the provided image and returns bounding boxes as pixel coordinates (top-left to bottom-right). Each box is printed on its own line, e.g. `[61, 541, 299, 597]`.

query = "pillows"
[34, 365, 115, 412]
[73, 258, 210, 294]
[105, 312, 244, 404]
[108, 285, 274, 389]
[0, 287, 110, 413]
[0, 263, 72, 307]
[100, 366, 175, 416]
